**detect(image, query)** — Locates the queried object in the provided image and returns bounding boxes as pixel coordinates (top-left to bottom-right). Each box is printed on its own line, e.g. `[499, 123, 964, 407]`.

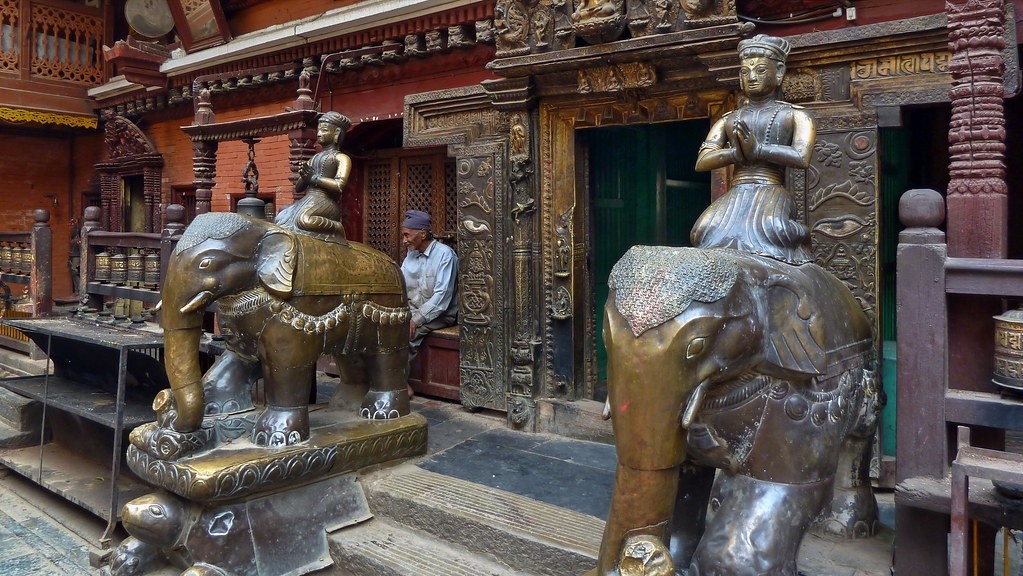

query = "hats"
[400, 209, 432, 232]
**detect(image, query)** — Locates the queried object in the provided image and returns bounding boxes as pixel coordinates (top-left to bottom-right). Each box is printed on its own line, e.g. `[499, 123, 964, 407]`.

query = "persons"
[67, 217, 85, 294]
[689, 33, 817, 266]
[274, 110, 353, 248]
[400, 210, 459, 399]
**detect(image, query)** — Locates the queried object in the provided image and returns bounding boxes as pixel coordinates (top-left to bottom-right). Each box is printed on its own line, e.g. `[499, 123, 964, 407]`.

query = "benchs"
[325, 322, 461, 403]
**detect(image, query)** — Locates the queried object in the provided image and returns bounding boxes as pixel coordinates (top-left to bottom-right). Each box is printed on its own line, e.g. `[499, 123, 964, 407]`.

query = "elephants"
[151, 213, 413, 447]
[596, 246, 881, 576]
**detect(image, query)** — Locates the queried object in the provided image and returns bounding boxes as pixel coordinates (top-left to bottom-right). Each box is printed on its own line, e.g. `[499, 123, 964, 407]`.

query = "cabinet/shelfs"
[1, 319, 209, 546]
